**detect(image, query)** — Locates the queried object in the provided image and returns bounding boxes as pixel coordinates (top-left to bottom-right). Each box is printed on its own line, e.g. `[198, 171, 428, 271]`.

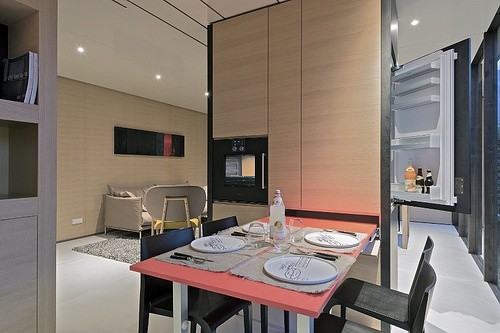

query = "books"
[4, 51, 38, 104]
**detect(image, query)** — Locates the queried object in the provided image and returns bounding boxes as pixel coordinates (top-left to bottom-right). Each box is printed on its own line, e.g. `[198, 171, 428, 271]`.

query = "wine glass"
[248, 223, 265, 265]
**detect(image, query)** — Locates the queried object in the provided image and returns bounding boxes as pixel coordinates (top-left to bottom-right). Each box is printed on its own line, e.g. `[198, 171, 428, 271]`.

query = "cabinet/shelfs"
[207, 0, 381, 226]
[0, 0, 56, 333]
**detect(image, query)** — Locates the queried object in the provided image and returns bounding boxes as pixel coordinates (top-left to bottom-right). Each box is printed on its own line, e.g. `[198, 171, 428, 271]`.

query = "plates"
[263, 255, 338, 285]
[304, 232, 360, 248]
[243, 223, 288, 235]
[190, 236, 246, 253]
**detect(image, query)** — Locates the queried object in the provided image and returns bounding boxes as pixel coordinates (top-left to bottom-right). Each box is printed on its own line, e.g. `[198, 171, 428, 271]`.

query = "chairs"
[145, 184, 206, 238]
[314, 236, 437, 333]
[137, 216, 289, 333]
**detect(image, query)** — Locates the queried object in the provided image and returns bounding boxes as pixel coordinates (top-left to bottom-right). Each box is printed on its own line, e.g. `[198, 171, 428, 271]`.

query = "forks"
[297, 248, 338, 258]
[170, 254, 208, 264]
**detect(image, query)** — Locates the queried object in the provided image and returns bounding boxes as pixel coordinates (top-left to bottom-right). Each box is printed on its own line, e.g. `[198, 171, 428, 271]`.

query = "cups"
[289, 219, 303, 242]
[273, 226, 291, 251]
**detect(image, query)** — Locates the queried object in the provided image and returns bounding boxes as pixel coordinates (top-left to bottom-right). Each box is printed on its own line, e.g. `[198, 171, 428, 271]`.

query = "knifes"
[174, 252, 214, 262]
[290, 252, 336, 260]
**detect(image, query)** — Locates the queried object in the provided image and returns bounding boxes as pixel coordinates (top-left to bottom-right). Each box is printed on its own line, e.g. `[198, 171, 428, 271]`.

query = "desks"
[129, 216, 377, 333]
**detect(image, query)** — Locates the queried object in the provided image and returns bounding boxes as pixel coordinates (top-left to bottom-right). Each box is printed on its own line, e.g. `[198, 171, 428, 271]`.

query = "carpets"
[72, 233, 151, 264]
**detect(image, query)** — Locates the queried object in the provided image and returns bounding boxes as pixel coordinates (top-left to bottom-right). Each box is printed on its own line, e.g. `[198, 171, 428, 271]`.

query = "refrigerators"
[391, 38, 471, 291]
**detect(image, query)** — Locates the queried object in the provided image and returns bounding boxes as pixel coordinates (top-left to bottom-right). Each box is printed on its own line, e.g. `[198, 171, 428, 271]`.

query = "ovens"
[212, 138, 268, 205]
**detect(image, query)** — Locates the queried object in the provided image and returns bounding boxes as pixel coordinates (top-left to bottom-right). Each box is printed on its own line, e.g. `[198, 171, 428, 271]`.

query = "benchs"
[104, 181, 207, 239]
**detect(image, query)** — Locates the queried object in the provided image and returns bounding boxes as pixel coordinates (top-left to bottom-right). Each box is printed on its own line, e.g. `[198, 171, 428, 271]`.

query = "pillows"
[114, 191, 147, 213]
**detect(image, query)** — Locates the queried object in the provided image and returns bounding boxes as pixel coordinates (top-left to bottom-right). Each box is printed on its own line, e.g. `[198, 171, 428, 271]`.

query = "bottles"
[416, 167, 424, 193]
[405, 160, 416, 193]
[269, 189, 285, 240]
[425, 169, 434, 194]
